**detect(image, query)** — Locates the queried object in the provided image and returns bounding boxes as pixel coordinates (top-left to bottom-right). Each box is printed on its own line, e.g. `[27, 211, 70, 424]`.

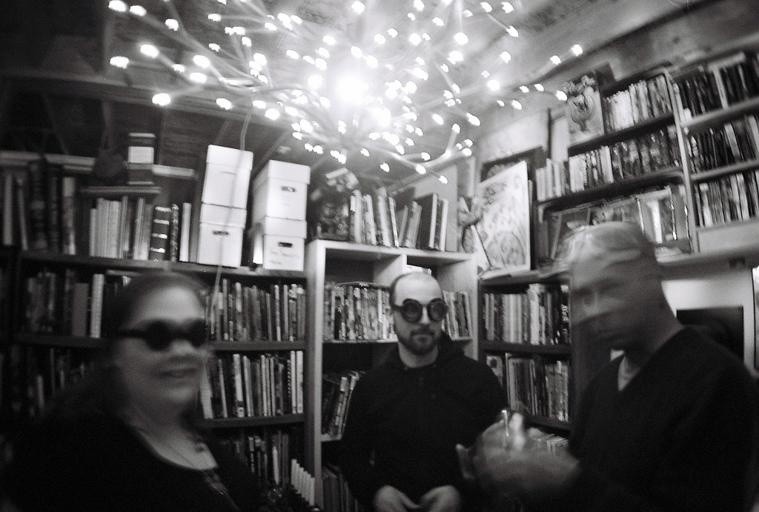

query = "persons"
[15, 268, 264, 507]
[478, 218, 758, 511]
[326, 272, 509, 509]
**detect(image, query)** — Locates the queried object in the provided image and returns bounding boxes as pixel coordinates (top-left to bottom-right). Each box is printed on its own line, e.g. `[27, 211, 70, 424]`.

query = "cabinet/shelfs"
[2, 154, 483, 511]
[478, 33, 757, 441]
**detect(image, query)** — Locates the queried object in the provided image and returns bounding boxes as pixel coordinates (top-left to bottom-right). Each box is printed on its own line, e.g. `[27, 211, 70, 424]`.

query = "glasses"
[112, 317, 211, 352]
[388, 301, 448, 324]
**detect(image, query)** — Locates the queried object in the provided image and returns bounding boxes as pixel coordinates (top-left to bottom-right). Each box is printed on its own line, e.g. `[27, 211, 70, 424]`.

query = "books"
[216, 426, 360, 511]
[0, 35, 759, 424]
